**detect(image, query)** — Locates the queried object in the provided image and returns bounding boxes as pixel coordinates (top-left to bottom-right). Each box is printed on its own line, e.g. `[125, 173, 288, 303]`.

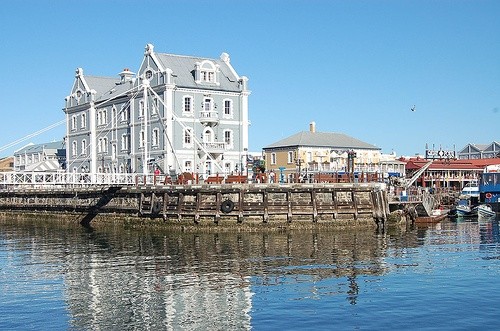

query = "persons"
[298, 169, 305, 183]
[154, 167, 161, 184]
[79, 166, 85, 183]
[269, 169, 275, 183]
[84, 165, 92, 183]
[388, 172, 482, 195]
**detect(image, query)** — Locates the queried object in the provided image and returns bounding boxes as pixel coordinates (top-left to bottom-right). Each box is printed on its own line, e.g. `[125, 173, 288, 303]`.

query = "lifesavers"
[486, 193, 492, 198]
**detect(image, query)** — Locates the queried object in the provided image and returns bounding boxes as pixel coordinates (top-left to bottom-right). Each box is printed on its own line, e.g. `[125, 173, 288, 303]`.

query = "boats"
[447, 199, 478, 218]
[476, 204, 495, 219]
